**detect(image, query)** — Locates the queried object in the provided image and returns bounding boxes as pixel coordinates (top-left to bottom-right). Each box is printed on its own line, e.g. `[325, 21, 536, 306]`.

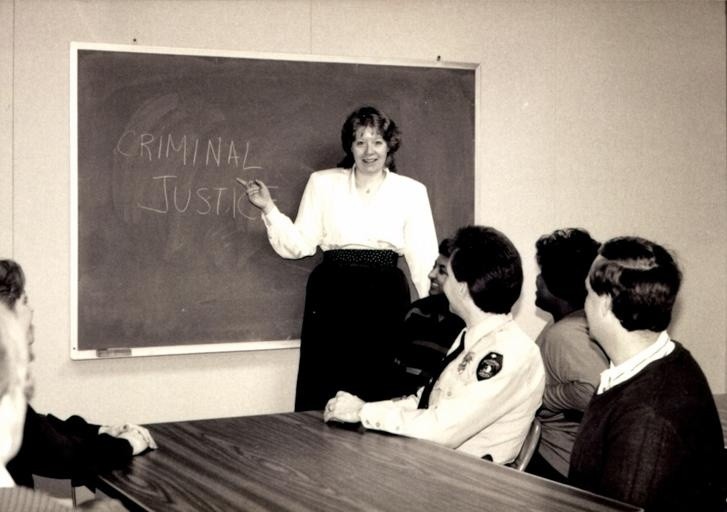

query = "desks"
[66, 405, 643, 512]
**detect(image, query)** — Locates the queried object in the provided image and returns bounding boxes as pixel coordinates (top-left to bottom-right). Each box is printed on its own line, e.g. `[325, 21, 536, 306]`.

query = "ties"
[415, 330, 467, 411]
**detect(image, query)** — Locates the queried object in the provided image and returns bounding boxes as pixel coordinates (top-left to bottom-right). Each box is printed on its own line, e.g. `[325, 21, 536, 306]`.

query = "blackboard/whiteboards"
[70, 40, 482, 360]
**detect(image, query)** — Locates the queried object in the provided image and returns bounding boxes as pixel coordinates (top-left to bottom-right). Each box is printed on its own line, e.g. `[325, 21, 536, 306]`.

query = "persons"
[322, 225, 546, 464]
[236, 107, 439, 412]
[566, 235, 727, 512]
[532, 227, 610, 483]
[0, 259, 157, 512]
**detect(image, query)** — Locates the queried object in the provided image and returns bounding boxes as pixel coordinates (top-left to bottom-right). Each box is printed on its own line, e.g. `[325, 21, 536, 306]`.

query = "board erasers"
[96, 347, 133, 359]
